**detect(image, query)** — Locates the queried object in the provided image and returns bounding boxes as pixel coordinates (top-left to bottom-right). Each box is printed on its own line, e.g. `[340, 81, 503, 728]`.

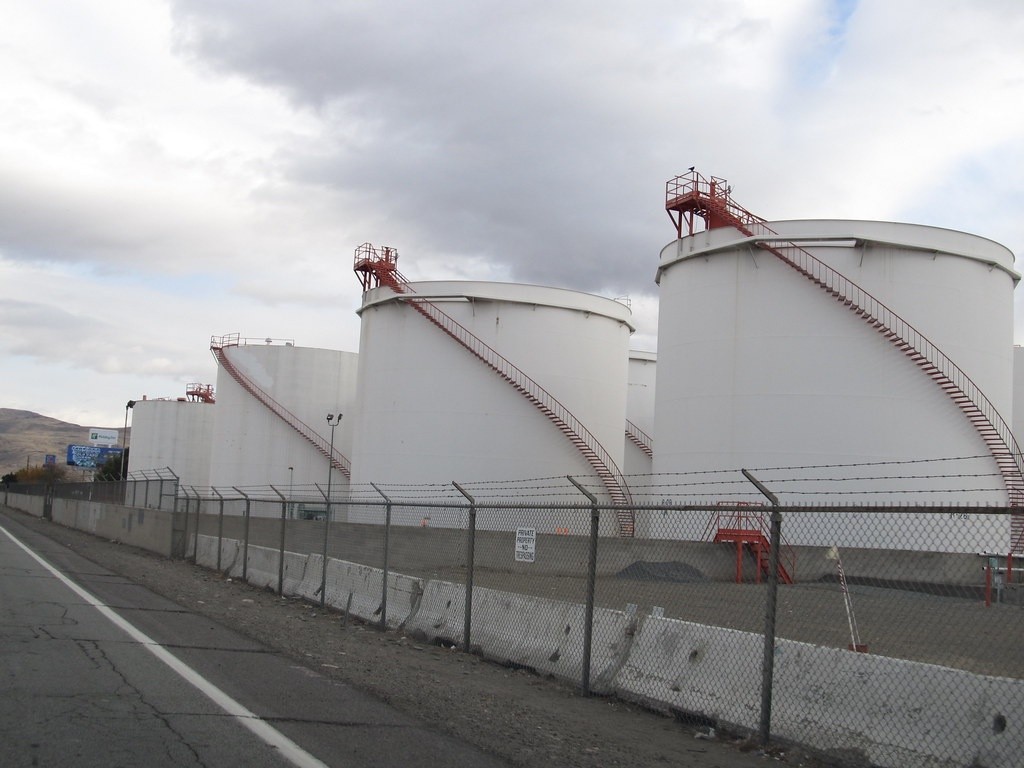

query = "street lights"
[120, 400, 136, 481]
[288, 466, 294, 501]
[326, 413, 344, 499]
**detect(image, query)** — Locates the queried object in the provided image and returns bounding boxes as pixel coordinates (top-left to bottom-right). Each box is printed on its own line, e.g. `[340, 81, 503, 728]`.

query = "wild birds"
[688, 166, 694, 171]
